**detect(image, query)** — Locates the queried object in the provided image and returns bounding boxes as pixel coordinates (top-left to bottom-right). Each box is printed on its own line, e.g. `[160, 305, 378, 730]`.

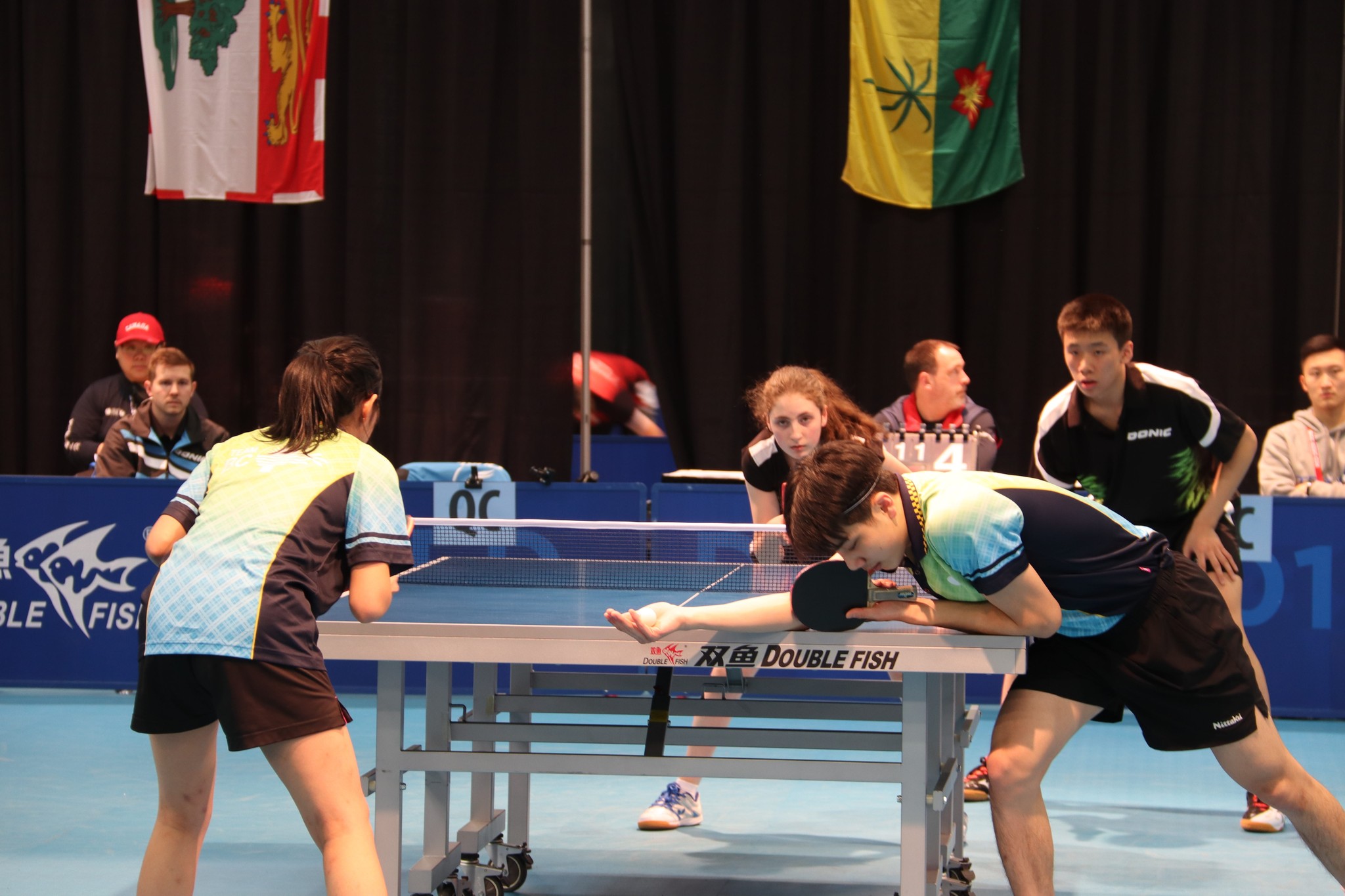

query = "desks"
[318, 560, 1030, 896]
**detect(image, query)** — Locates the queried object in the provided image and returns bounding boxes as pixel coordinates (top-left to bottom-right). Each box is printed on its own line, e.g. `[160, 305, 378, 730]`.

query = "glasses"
[118, 342, 160, 355]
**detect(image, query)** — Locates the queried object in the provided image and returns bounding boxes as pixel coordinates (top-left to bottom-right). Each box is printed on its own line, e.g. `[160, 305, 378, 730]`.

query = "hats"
[114, 311, 165, 347]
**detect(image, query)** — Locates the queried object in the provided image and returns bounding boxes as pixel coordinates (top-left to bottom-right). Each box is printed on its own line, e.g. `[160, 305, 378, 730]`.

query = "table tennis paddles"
[790, 560, 918, 633]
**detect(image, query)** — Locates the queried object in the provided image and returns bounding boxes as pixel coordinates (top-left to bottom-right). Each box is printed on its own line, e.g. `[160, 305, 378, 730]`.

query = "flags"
[840, 0, 1026, 210]
[136, 0, 329, 204]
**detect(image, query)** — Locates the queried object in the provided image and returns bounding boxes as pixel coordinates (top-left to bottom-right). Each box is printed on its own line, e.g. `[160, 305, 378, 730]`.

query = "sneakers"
[638, 782, 704, 829]
[1240, 791, 1285, 832]
[963, 755, 991, 800]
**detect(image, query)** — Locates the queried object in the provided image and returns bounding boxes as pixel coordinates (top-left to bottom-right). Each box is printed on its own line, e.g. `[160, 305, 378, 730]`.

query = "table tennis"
[638, 608, 656, 627]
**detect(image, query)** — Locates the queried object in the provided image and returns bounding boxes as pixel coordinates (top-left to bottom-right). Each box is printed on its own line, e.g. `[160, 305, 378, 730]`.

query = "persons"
[62, 311, 210, 478]
[634, 364, 912, 831]
[130, 333, 413, 896]
[1254, 333, 1345, 499]
[601, 439, 1345, 895]
[872, 338, 1003, 472]
[964, 292, 1287, 833]
[91, 346, 230, 478]
